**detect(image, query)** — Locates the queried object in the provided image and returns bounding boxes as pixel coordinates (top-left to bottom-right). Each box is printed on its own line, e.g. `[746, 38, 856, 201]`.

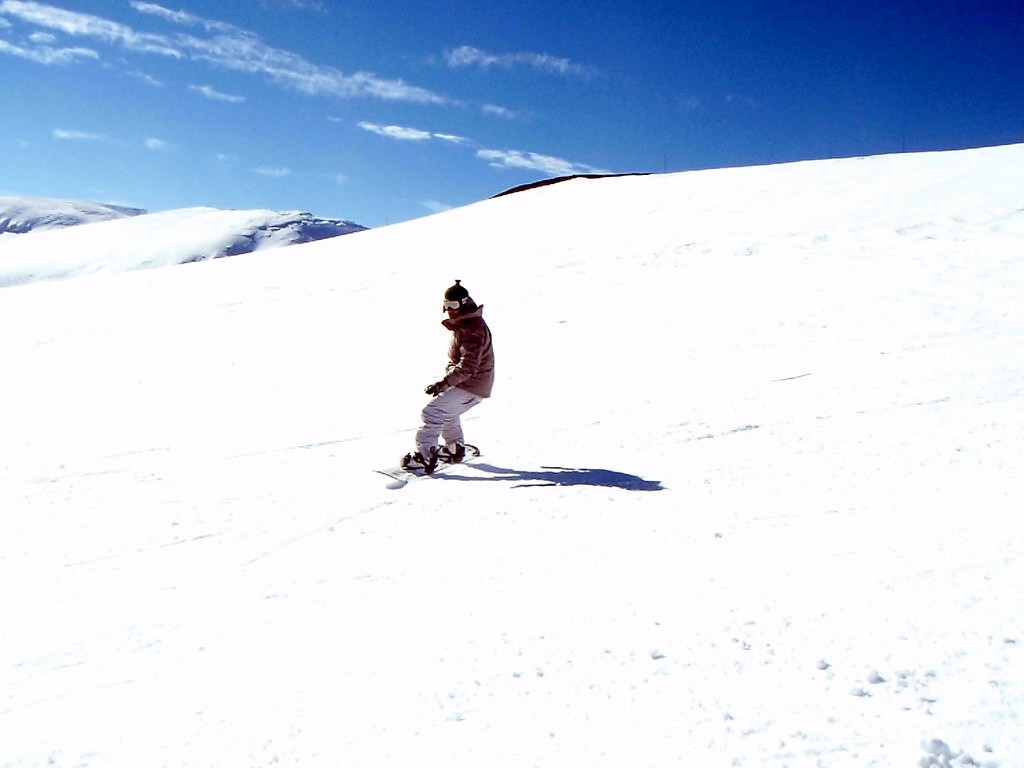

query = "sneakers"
[438, 443, 465, 464]
[400, 454, 438, 475]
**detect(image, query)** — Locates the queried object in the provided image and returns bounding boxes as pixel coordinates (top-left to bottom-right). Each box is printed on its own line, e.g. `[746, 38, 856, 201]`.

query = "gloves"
[425, 380, 451, 397]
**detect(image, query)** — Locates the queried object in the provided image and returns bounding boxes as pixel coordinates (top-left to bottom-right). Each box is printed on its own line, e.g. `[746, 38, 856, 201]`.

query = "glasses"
[443, 300, 459, 310]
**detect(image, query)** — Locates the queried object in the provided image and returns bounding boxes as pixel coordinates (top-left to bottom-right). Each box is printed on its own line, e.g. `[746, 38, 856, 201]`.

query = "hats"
[442, 279, 468, 310]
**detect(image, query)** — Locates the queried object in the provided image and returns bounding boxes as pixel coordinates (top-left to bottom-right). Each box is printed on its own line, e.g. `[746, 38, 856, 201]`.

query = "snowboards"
[372, 443, 480, 483]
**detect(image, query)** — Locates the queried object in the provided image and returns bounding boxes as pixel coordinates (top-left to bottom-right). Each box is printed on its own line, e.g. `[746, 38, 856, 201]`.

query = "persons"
[400, 280, 493, 479]
